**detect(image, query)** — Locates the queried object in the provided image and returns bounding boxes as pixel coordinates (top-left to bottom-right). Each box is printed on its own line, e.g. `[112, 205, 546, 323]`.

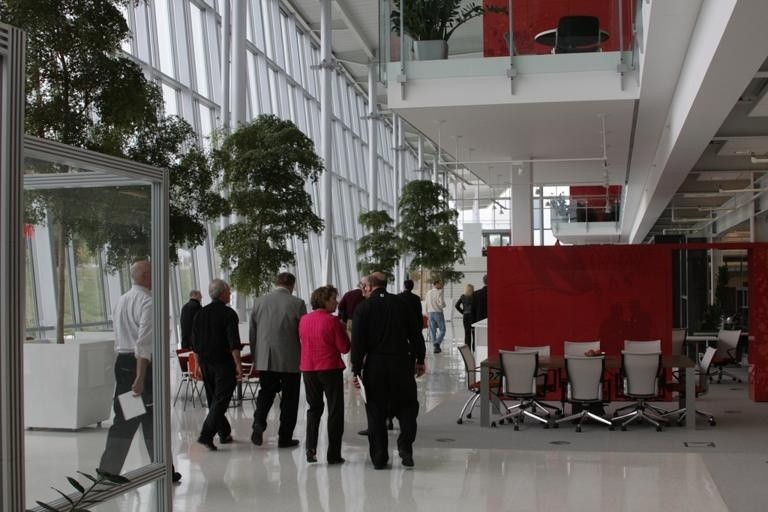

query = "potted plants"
[390, 0, 508, 60]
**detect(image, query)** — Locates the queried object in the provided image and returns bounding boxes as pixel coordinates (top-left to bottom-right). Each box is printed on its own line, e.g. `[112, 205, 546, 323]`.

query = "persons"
[246, 270, 309, 452]
[295, 282, 353, 467]
[189, 275, 245, 453]
[454, 282, 474, 350]
[470, 272, 489, 327]
[390, 273, 423, 320]
[97, 253, 182, 488]
[347, 268, 426, 475]
[337, 272, 368, 350]
[421, 277, 450, 352]
[179, 286, 203, 352]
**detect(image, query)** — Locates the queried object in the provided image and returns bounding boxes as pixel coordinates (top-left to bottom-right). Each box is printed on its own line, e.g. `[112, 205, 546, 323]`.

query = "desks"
[534, 28, 610, 48]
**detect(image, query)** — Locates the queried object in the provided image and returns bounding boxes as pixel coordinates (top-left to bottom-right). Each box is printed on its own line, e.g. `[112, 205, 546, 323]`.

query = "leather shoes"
[399, 449, 413, 466]
[199, 436, 232, 450]
[357, 428, 368, 436]
[252, 424, 262, 445]
[174, 472, 181, 482]
[278, 440, 345, 464]
[434, 343, 441, 353]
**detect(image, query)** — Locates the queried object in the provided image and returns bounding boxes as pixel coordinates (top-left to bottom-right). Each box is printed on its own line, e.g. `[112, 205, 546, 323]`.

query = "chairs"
[456, 327, 744, 432]
[172, 349, 260, 411]
[554, 16, 602, 54]
